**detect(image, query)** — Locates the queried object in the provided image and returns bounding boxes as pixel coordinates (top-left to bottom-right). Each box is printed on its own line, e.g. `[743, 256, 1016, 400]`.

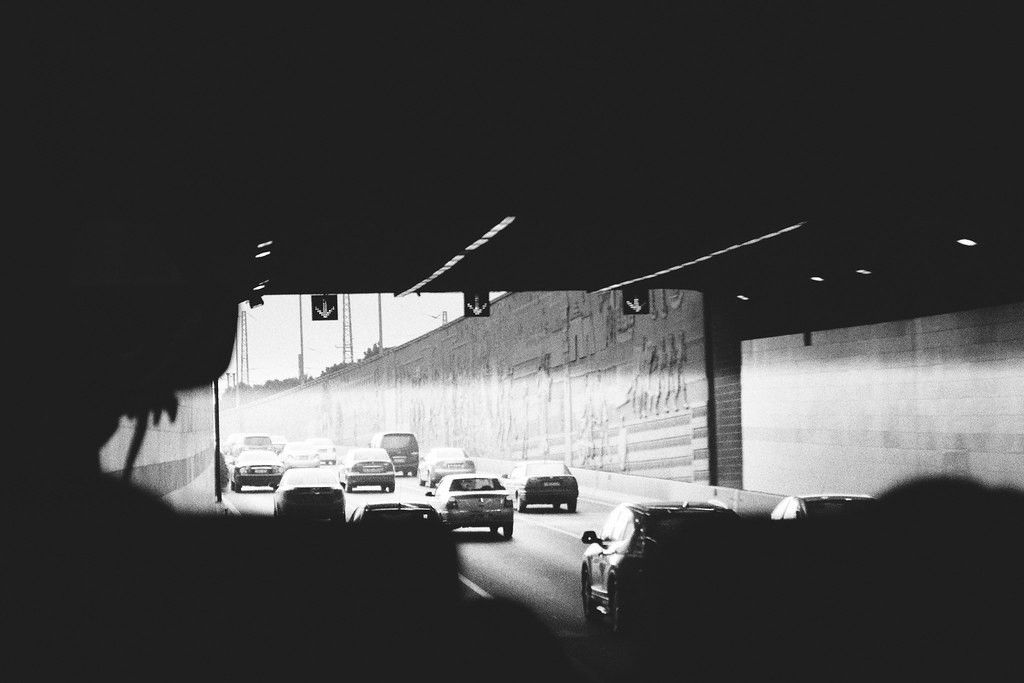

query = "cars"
[503, 458, 579, 514]
[577, 492, 903, 647]
[269, 467, 346, 523]
[306, 438, 338, 465]
[419, 448, 476, 494]
[425, 472, 515, 538]
[228, 450, 285, 493]
[339, 446, 396, 494]
[280, 441, 322, 471]
[348, 500, 453, 538]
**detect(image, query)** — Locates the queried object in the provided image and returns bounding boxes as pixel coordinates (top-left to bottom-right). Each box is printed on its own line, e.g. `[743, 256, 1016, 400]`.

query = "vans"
[235, 434, 274, 452]
[370, 431, 420, 477]
